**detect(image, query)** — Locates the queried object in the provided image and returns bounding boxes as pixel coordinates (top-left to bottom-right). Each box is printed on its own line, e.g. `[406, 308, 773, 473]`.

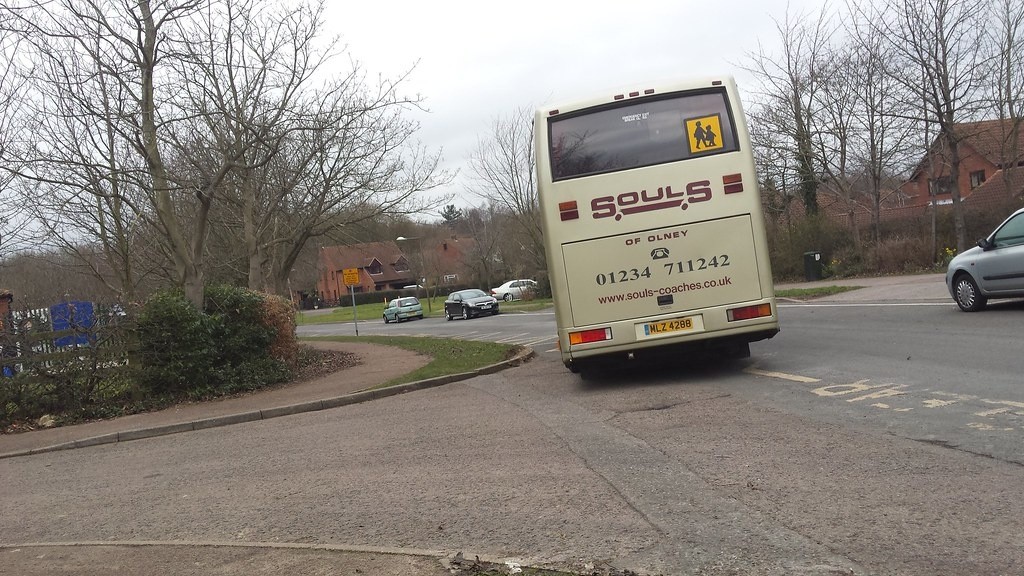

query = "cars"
[403, 285, 422, 289]
[946, 207, 1024, 312]
[444, 289, 499, 321]
[383, 297, 423, 323]
[489, 279, 539, 302]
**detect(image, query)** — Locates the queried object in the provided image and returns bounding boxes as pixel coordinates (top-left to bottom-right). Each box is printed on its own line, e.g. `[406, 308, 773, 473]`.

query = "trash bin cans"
[803, 251, 823, 282]
[311, 296, 319, 309]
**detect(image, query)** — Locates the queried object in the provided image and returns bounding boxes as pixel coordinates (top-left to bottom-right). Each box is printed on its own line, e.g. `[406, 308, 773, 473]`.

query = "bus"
[532, 74, 780, 373]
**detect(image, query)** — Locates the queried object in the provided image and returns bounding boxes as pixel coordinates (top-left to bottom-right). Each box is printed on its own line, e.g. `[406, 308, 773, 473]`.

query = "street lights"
[397, 236, 432, 313]
